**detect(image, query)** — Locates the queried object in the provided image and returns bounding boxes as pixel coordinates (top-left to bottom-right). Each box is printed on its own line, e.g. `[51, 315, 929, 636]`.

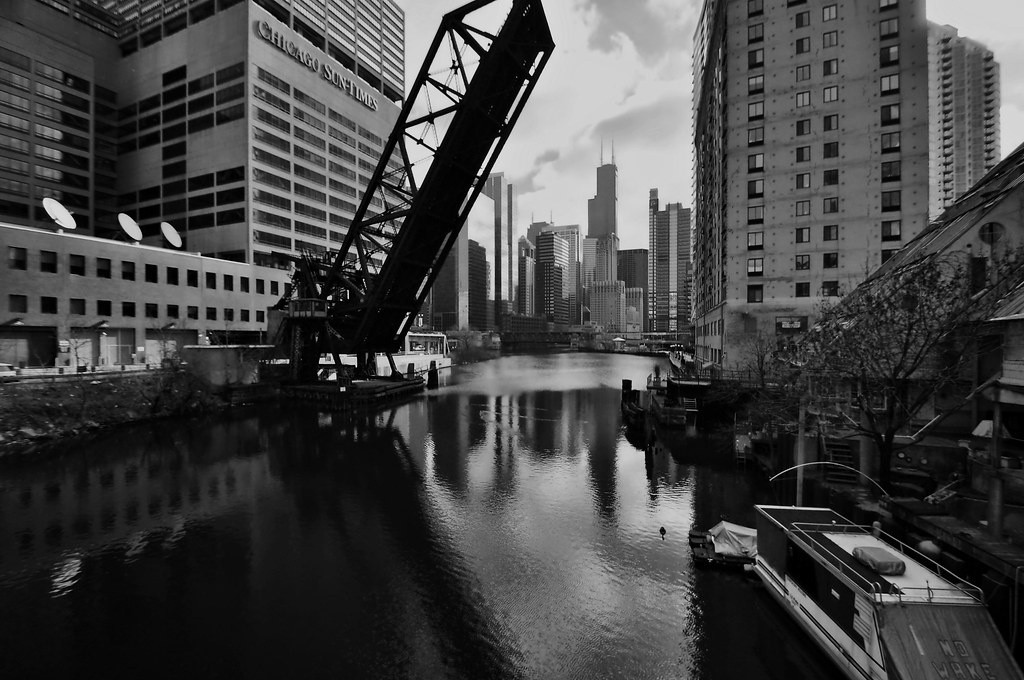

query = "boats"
[318, 329, 452, 377]
[621, 399, 649, 428]
[689, 529, 756, 568]
[750, 505, 1024, 680]
